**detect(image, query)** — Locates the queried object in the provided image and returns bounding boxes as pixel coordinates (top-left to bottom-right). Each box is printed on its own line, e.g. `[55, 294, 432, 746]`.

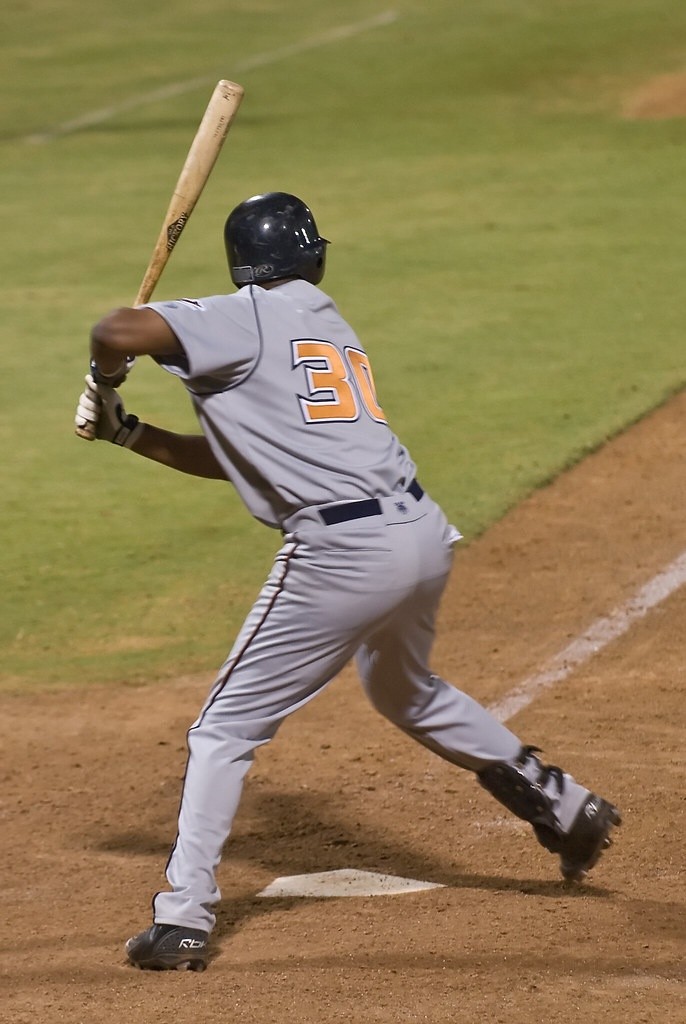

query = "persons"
[74, 192, 623, 973]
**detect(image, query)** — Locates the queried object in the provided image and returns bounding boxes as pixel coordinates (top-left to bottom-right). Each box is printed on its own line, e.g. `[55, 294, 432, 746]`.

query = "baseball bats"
[73, 73, 246, 444]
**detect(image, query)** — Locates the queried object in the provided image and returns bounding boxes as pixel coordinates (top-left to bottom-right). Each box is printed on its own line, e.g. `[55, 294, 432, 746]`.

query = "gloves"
[89, 354, 136, 388]
[75, 375, 142, 449]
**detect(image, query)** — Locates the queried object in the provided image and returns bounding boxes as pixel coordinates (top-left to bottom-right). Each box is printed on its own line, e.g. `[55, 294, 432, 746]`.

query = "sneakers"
[559, 794, 623, 883]
[124, 924, 211, 971]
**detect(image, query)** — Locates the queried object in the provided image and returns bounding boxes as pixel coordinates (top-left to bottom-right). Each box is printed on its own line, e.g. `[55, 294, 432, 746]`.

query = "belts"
[318, 478, 425, 526]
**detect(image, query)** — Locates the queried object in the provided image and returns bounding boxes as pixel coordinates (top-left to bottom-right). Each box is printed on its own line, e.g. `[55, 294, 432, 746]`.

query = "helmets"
[223, 190, 331, 288]
